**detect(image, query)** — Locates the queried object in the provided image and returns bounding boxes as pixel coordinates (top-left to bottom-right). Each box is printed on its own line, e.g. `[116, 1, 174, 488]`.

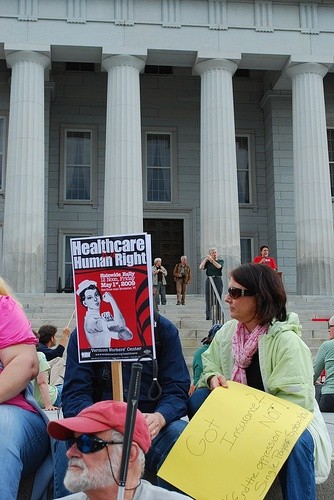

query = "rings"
[153, 430, 156, 434]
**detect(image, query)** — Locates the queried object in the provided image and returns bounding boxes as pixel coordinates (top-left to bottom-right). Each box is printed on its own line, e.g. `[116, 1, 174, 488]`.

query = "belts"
[211, 276, 221, 278]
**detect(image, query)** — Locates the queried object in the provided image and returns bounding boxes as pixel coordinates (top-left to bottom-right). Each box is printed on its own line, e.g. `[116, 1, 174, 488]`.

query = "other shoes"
[176, 303, 185, 305]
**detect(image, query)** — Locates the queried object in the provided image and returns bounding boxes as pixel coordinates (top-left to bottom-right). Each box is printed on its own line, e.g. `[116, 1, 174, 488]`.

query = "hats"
[77, 280, 97, 296]
[46, 399, 151, 454]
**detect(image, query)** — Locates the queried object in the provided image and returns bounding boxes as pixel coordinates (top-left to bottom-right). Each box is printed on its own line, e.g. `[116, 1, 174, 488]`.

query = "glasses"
[327, 322, 334, 329]
[228, 287, 254, 299]
[208, 324, 222, 338]
[70, 432, 123, 454]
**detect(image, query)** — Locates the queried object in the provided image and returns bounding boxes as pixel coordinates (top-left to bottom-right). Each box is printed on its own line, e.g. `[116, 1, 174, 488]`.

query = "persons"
[151, 248, 224, 320]
[0, 275, 223, 500]
[252, 246, 277, 271]
[188, 262, 332, 500]
[313, 316, 334, 413]
